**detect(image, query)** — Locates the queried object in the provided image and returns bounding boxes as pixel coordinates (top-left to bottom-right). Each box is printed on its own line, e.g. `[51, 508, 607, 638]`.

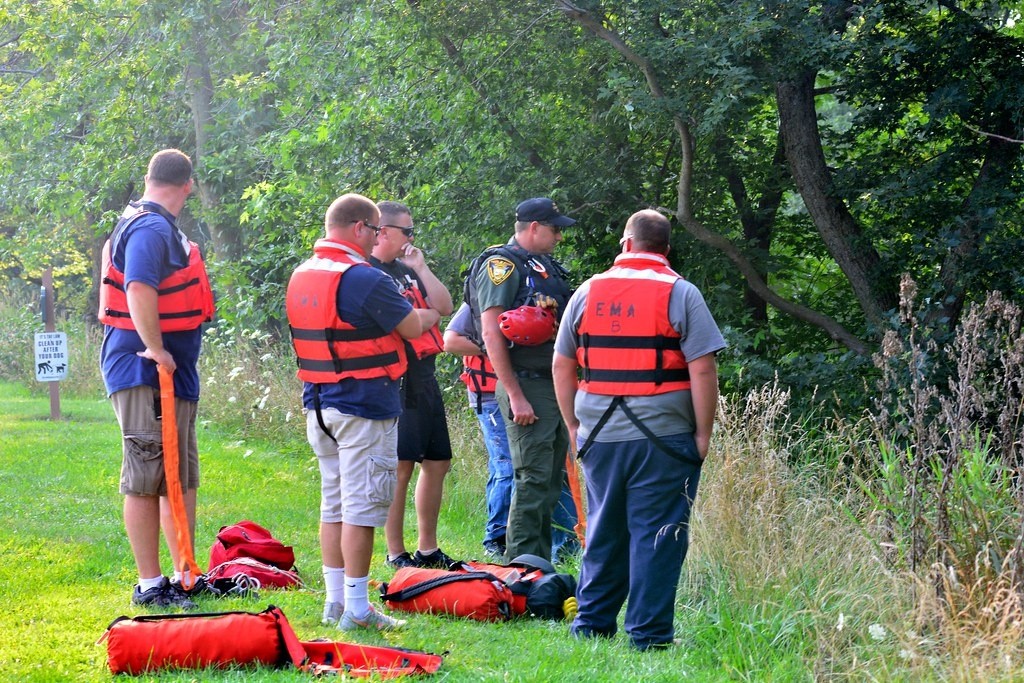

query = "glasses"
[379, 224, 414, 236]
[350, 220, 381, 236]
[554, 226, 562, 233]
[619, 234, 635, 244]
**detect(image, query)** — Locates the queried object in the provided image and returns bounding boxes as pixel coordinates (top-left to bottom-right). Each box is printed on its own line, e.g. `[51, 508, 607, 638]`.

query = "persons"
[97, 149, 214, 611]
[551, 209, 726, 653]
[285, 193, 576, 632]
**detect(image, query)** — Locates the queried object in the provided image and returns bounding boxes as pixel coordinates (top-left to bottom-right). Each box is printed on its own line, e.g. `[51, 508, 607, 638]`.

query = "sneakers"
[322, 601, 407, 632]
[485, 542, 505, 557]
[170, 576, 220, 599]
[131, 576, 198, 612]
[384, 548, 457, 571]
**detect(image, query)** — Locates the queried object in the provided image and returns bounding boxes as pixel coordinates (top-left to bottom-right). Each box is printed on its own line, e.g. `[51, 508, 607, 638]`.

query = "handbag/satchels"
[385, 553, 578, 621]
[108, 604, 284, 672]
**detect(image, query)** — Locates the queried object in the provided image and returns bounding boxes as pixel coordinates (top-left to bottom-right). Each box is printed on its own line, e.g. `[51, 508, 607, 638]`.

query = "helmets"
[497, 305, 554, 346]
[516, 197, 576, 226]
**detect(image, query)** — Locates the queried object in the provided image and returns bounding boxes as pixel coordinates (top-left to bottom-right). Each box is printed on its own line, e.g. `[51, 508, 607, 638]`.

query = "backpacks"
[206, 521, 299, 589]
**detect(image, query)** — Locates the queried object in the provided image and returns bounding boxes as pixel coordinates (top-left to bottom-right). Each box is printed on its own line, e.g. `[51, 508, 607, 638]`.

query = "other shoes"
[630, 639, 698, 655]
